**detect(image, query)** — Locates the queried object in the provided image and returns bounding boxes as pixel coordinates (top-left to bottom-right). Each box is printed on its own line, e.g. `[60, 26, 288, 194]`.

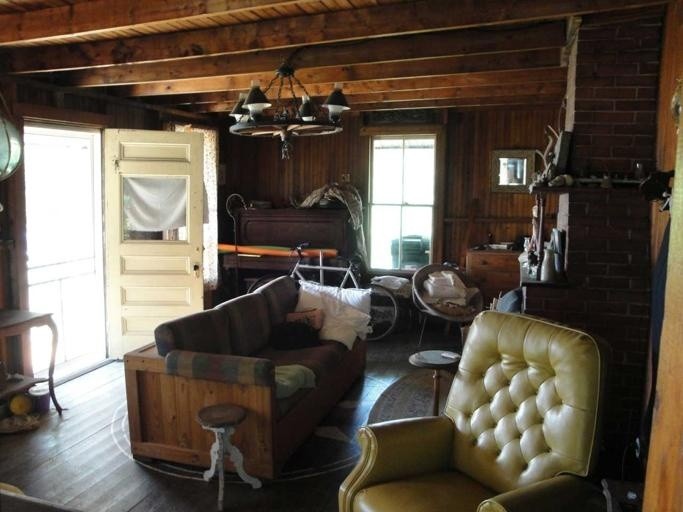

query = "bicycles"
[243, 241, 399, 344]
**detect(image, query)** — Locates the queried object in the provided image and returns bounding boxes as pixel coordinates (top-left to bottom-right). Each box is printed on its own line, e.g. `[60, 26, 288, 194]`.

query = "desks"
[405, 348, 460, 417]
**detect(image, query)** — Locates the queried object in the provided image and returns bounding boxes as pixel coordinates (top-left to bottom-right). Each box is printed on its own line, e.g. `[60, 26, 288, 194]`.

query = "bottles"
[522, 237, 530, 252]
[539, 249, 555, 283]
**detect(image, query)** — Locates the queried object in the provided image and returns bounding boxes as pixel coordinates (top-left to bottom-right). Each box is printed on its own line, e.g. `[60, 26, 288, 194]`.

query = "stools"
[195, 402, 263, 511]
[244, 276, 271, 293]
[0, 309, 62, 423]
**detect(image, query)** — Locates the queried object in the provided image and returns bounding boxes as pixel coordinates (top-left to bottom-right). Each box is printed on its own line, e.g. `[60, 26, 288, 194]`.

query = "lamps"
[227, 45, 349, 163]
[0, 99, 27, 215]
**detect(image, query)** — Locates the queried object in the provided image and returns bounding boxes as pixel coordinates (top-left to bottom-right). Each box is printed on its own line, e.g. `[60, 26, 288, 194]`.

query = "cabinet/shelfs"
[464, 246, 520, 309]
[516, 171, 571, 290]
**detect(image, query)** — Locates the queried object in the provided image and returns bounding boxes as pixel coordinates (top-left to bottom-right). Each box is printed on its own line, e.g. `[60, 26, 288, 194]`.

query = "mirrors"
[489, 147, 535, 196]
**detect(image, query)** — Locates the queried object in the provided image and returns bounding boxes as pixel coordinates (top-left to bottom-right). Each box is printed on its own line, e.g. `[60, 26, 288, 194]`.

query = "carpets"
[365, 369, 452, 424]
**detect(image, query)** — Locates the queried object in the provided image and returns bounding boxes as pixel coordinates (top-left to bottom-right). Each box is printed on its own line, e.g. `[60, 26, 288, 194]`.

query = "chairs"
[411, 261, 483, 347]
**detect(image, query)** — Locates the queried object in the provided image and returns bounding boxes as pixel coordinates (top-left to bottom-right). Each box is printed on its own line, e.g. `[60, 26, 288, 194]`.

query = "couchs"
[336, 308, 600, 512]
[117, 274, 367, 480]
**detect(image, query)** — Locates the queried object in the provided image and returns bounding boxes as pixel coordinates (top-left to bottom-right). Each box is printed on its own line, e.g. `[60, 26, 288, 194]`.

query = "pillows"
[317, 292, 371, 350]
[294, 279, 373, 334]
